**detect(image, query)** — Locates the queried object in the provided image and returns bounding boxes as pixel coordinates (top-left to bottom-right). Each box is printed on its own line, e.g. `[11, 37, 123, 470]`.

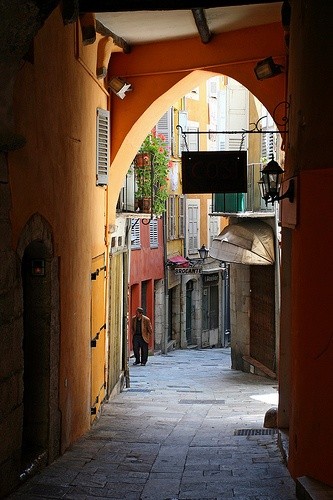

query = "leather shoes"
[141, 363, 145, 366]
[133, 361, 139, 365]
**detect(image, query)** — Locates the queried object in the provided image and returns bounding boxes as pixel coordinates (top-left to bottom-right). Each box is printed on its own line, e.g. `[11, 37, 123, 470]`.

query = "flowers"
[133, 126, 174, 219]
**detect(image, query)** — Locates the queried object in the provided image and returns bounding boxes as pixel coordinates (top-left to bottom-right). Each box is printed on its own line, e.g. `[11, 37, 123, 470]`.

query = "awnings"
[208, 221, 274, 266]
[169, 253, 191, 270]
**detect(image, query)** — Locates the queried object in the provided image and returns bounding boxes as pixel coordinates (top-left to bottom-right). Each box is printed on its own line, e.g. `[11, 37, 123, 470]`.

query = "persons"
[131, 307, 153, 367]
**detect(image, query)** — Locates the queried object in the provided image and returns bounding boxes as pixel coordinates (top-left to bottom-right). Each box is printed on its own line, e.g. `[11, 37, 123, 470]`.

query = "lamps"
[253, 56, 286, 82]
[107, 75, 134, 100]
[185, 243, 210, 268]
[258, 175, 280, 208]
[259, 153, 295, 204]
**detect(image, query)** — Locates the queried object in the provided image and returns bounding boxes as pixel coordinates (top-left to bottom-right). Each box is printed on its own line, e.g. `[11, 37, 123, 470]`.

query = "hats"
[136, 307, 143, 312]
